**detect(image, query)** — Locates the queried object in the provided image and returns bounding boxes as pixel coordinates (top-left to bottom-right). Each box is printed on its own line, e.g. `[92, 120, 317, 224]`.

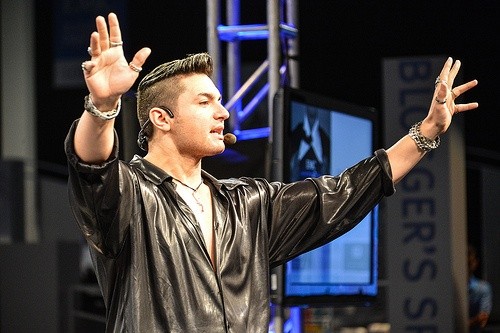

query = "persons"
[291, 102, 331, 177]
[64, 12, 479, 333]
[467, 242, 492, 333]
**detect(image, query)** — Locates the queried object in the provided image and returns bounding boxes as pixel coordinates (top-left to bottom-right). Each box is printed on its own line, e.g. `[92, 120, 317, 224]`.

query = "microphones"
[224, 133, 237, 145]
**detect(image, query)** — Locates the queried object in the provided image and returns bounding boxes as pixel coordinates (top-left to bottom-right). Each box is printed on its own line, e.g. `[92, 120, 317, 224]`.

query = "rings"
[87, 47, 101, 57]
[129, 61, 142, 72]
[435, 76, 447, 85]
[451, 90, 458, 98]
[81, 61, 92, 72]
[110, 41, 123, 47]
[435, 96, 447, 104]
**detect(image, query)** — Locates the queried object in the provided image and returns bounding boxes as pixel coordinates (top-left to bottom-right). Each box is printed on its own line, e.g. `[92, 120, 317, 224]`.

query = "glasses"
[138, 106, 174, 151]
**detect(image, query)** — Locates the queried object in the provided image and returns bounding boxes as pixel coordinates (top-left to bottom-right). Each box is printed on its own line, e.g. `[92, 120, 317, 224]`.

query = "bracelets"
[408, 120, 440, 152]
[84, 92, 122, 121]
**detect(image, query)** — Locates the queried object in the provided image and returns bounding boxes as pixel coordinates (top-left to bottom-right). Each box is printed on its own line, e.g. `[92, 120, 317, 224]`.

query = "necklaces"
[171, 177, 205, 212]
